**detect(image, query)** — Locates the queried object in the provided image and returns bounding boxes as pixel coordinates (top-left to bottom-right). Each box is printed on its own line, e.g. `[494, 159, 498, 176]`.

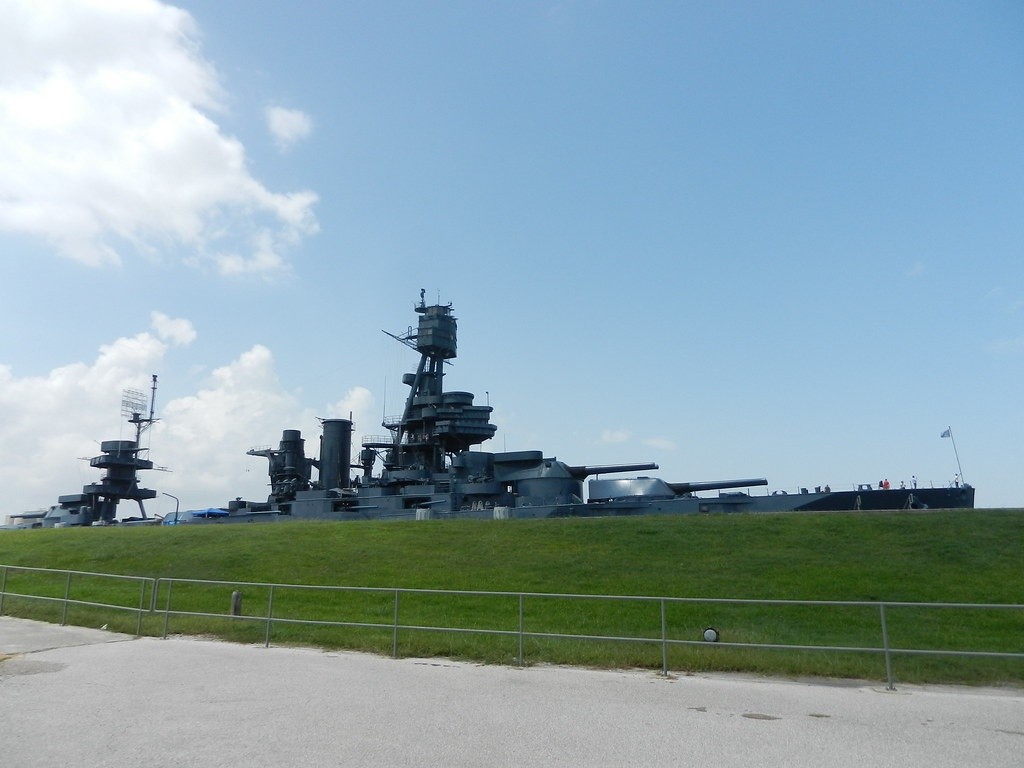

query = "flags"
[940, 429, 950, 438]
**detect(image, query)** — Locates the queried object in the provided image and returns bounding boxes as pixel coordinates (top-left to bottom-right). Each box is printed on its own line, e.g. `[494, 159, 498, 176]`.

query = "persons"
[900, 481, 906, 489]
[954, 474, 959, 487]
[912, 476, 917, 489]
[825, 485, 830, 491]
[883, 479, 889, 489]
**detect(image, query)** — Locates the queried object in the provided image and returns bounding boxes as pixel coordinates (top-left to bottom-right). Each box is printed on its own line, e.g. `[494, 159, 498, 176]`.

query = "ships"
[4, 287, 975, 529]
